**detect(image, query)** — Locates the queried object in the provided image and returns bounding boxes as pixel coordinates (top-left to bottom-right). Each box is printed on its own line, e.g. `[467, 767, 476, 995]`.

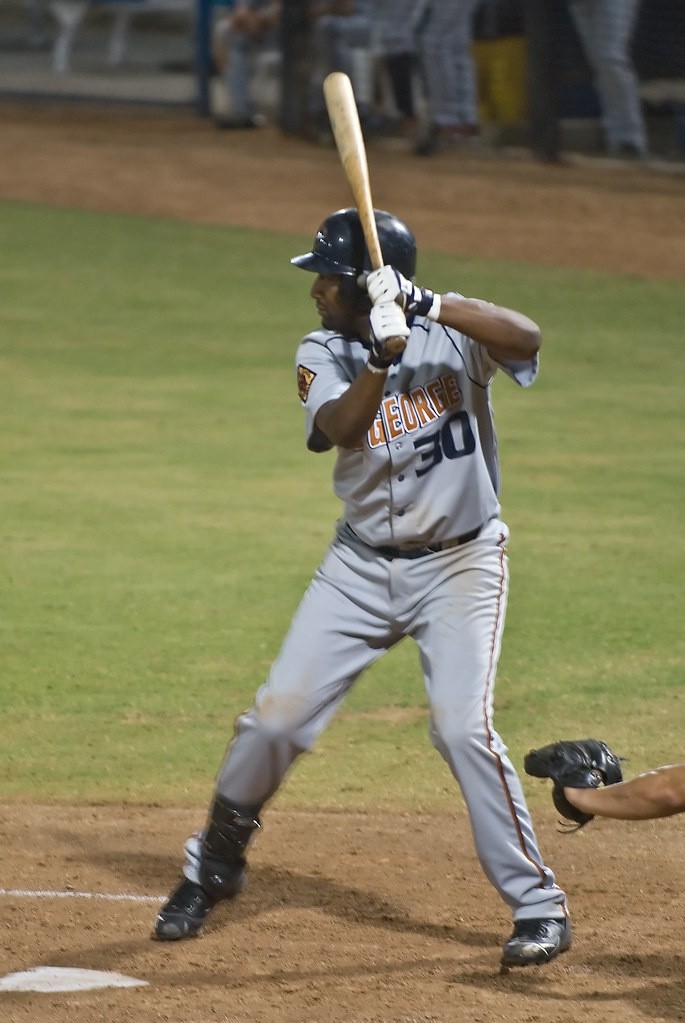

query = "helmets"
[290, 207, 417, 284]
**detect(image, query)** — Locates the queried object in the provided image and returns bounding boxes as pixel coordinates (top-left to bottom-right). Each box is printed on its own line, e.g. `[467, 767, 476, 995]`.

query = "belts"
[345, 515, 498, 561]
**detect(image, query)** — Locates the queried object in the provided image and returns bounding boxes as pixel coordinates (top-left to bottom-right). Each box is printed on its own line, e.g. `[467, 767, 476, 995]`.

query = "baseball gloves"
[522, 737, 630, 837]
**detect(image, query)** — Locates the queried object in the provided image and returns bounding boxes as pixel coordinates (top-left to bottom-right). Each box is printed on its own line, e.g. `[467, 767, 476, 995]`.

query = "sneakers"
[502, 916, 572, 965]
[154, 794, 263, 939]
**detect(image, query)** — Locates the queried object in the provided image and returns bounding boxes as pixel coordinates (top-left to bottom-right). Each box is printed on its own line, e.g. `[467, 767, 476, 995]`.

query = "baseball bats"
[322, 71, 409, 356]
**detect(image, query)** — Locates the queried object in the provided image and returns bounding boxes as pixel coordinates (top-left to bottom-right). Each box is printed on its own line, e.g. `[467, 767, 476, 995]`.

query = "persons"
[216, 0, 647, 163]
[157, 207, 574, 967]
[525, 738, 685, 826]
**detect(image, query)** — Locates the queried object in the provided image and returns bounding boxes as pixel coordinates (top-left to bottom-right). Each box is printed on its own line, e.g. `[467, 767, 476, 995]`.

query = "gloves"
[365, 265, 442, 322]
[367, 303, 410, 374]
